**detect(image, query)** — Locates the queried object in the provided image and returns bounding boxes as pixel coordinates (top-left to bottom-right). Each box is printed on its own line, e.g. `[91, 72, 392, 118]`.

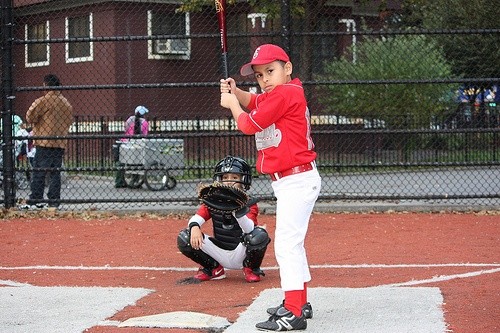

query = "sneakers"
[256, 307, 307, 331]
[267, 301, 314, 318]
[194, 265, 226, 281]
[242, 267, 261, 282]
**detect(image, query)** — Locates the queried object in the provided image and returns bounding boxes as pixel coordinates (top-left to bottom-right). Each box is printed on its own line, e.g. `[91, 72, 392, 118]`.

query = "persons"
[20, 74, 74, 208]
[221, 45, 321, 333]
[113, 106, 149, 189]
[176, 156, 271, 282]
[13, 121, 36, 170]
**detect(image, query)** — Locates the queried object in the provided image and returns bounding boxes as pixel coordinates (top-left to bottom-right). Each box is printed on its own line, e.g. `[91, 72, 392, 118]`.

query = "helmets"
[215, 157, 252, 192]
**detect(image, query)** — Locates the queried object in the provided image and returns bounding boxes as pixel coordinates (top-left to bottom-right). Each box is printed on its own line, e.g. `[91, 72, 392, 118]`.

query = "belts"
[270, 163, 312, 181]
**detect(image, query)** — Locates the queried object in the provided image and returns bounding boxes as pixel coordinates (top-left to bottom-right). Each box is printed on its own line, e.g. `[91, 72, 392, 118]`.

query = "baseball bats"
[214, 0, 231, 94]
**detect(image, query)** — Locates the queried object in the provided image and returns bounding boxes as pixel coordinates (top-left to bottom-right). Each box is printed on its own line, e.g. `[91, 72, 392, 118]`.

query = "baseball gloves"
[197, 181, 251, 213]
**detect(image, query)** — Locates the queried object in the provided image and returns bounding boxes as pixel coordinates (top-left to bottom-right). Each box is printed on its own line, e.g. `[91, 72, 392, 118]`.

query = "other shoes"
[19, 203, 43, 210]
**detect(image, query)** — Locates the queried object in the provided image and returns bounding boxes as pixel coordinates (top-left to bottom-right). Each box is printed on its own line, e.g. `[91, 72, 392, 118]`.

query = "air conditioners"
[157, 35, 187, 53]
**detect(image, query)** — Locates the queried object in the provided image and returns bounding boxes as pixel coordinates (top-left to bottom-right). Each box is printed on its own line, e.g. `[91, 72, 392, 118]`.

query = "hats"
[135, 106, 149, 116]
[241, 44, 289, 76]
